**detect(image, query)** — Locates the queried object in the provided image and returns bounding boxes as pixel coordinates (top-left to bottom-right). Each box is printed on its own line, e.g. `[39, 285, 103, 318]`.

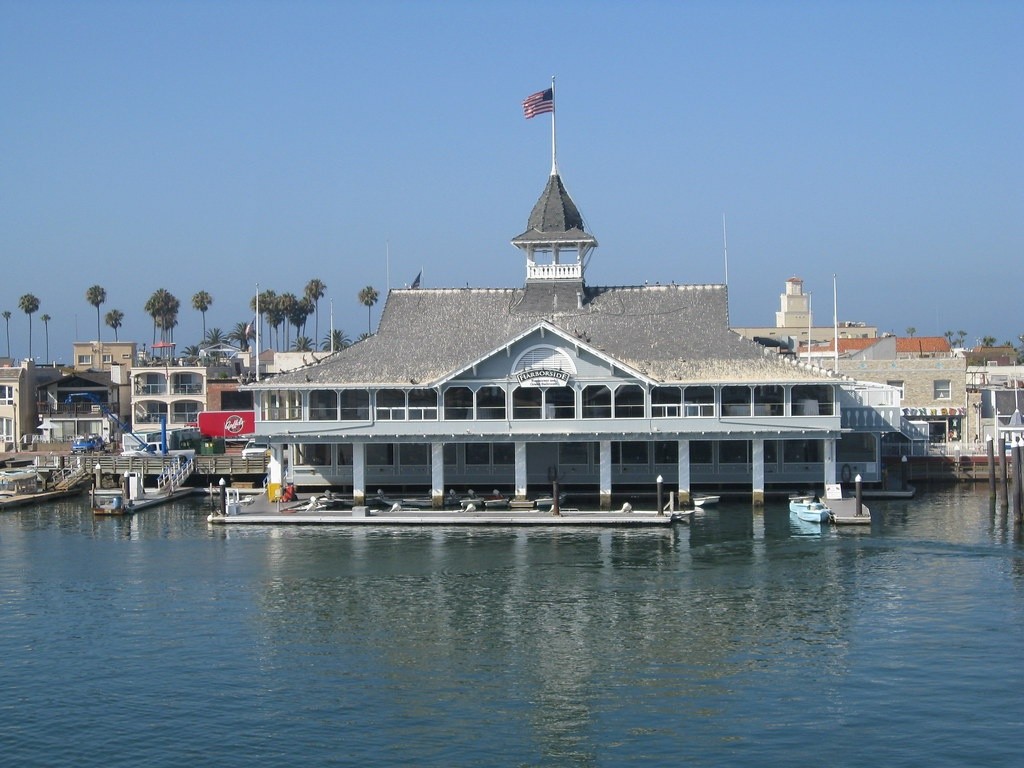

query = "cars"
[242, 439, 267, 460]
[72, 433, 103, 453]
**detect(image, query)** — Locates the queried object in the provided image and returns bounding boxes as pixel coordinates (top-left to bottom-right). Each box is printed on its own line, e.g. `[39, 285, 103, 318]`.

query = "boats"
[693, 495, 720, 508]
[0, 467, 43, 500]
[789, 499, 822, 513]
[294, 489, 567, 511]
[226, 496, 257, 506]
[797, 508, 831, 523]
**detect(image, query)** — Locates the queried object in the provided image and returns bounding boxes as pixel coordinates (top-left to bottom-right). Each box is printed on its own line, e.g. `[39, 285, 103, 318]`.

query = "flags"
[523, 86, 554, 120]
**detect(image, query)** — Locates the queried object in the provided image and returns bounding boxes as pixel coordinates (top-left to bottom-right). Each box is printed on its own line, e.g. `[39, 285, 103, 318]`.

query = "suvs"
[121, 441, 169, 456]
